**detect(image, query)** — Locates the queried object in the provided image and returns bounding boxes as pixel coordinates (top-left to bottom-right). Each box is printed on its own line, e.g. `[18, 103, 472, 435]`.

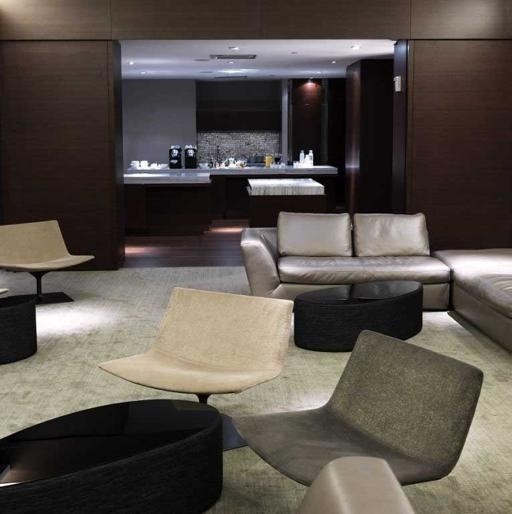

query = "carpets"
[0, 266, 512, 513]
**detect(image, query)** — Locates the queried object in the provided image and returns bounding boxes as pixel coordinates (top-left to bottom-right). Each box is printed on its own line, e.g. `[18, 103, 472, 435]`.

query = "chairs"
[230, 328, 482, 490]
[98, 285, 295, 453]
[0, 219, 95, 305]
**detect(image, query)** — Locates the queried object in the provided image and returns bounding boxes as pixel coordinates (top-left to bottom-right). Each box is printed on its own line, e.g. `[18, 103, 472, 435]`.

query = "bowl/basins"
[199, 163, 208, 169]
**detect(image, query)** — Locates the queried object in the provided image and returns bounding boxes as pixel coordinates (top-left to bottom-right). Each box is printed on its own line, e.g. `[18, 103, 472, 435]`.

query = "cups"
[169, 145, 196, 168]
[265, 153, 283, 167]
[130, 161, 157, 169]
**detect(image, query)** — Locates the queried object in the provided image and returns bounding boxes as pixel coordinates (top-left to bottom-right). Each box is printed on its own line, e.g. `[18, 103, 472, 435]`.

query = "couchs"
[239, 209, 451, 309]
[433, 246, 511, 354]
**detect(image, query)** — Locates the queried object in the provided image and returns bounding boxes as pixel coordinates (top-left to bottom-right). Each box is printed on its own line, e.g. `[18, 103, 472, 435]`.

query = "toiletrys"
[309, 150, 313, 162]
[300, 150, 305, 163]
[305, 155, 309, 164]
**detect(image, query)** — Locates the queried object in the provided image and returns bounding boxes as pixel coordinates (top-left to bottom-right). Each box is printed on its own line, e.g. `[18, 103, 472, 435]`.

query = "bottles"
[293, 150, 314, 168]
